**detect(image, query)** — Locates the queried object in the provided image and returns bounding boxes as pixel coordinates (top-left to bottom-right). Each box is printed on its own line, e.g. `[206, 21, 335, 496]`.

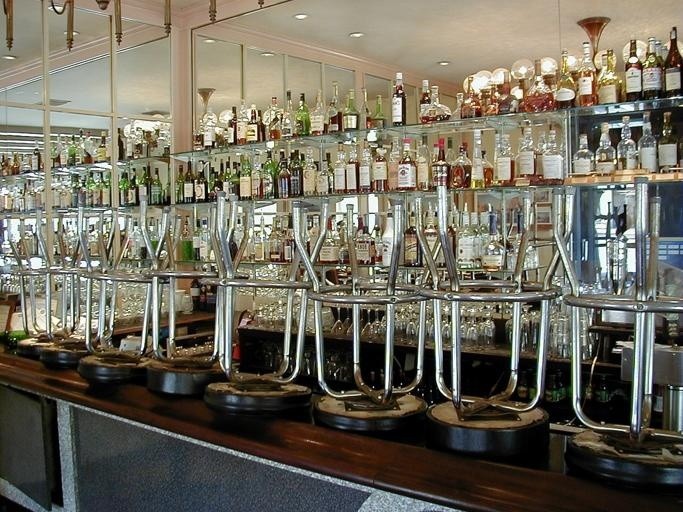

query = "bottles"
[268, 97, 283, 141]
[211, 171, 223, 201]
[283, 213, 296, 264]
[290, 149, 301, 198]
[120, 168, 129, 206]
[498, 71, 510, 111]
[0, 153, 28, 175]
[354, 215, 367, 265]
[126, 217, 147, 262]
[60, 147, 69, 168]
[209, 166, 215, 189]
[403, 211, 422, 265]
[359, 89, 372, 132]
[598, 49, 620, 105]
[76, 147, 82, 166]
[125, 119, 136, 160]
[451, 93, 464, 121]
[446, 137, 456, 166]
[309, 214, 321, 255]
[199, 283, 207, 312]
[237, 100, 250, 146]
[392, 72, 407, 128]
[470, 128, 486, 190]
[139, 167, 150, 203]
[334, 140, 348, 195]
[97, 130, 109, 162]
[490, 84, 498, 114]
[422, 85, 452, 123]
[541, 73, 555, 92]
[518, 119, 537, 186]
[328, 81, 343, 133]
[450, 145, 472, 190]
[482, 87, 490, 115]
[194, 159, 208, 204]
[480, 149, 494, 189]
[146, 165, 152, 187]
[183, 161, 195, 204]
[473, 93, 482, 117]
[556, 51, 576, 109]
[127, 166, 139, 204]
[461, 75, 475, 118]
[513, 78, 532, 101]
[274, 149, 284, 196]
[200, 226, 213, 261]
[262, 151, 275, 178]
[419, 79, 431, 124]
[507, 213, 538, 269]
[480, 213, 505, 271]
[663, 27, 683, 97]
[577, 42, 596, 108]
[117, 127, 124, 161]
[625, 39, 643, 103]
[164, 168, 174, 204]
[206, 284, 215, 311]
[595, 373, 611, 404]
[422, 209, 439, 267]
[430, 143, 441, 168]
[595, 122, 616, 173]
[573, 133, 595, 173]
[295, 92, 311, 137]
[239, 153, 252, 200]
[200, 106, 216, 147]
[397, 139, 416, 193]
[363, 225, 371, 265]
[370, 236, 377, 266]
[246, 104, 259, 144]
[223, 161, 232, 183]
[382, 208, 396, 266]
[338, 222, 350, 264]
[372, 148, 389, 193]
[68, 134, 77, 167]
[61, 135, 70, 152]
[192, 226, 203, 261]
[9, 216, 108, 262]
[617, 117, 637, 171]
[218, 161, 227, 190]
[148, 217, 159, 251]
[270, 218, 284, 262]
[189, 276, 200, 314]
[433, 139, 451, 189]
[515, 138, 525, 186]
[347, 89, 359, 130]
[359, 139, 373, 194]
[557, 372, 567, 398]
[517, 371, 529, 400]
[56, 135, 62, 155]
[319, 218, 341, 265]
[257, 109, 266, 144]
[547, 372, 560, 402]
[250, 152, 265, 198]
[52, 142, 61, 168]
[496, 135, 515, 188]
[542, 129, 564, 186]
[417, 140, 431, 192]
[84, 131, 93, 146]
[528, 373, 538, 401]
[658, 112, 679, 174]
[2, 171, 112, 212]
[387, 135, 403, 193]
[341, 90, 352, 132]
[179, 221, 194, 262]
[346, 139, 359, 195]
[447, 210, 456, 262]
[524, 59, 555, 112]
[176, 166, 186, 204]
[315, 159, 334, 195]
[656, 41, 662, 70]
[325, 153, 334, 195]
[134, 134, 144, 160]
[32, 139, 40, 172]
[638, 110, 657, 174]
[535, 127, 549, 186]
[230, 162, 240, 197]
[375, 226, 382, 265]
[281, 89, 296, 138]
[150, 167, 163, 205]
[596, 55, 608, 87]
[303, 145, 317, 197]
[308, 89, 328, 136]
[254, 215, 270, 261]
[300, 152, 304, 167]
[228, 106, 239, 146]
[641, 36, 661, 100]
[374, 95, 388, 130]
[277, 158, 290, 200]
[77, 129, 85, 164]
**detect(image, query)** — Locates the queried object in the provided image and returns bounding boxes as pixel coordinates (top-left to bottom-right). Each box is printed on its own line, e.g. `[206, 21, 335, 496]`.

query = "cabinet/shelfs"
[171, 96, 681, 369]
[0, 154, 169, 295]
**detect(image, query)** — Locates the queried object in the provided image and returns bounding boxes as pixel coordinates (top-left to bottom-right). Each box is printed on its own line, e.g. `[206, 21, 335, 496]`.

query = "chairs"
[8, 214, 91, 357]
[32, 205, 132, 366]
[552, 176, 682, 486]
[201, 191, 329, 411]
[412, 172, 577, 455]
[292, 191, 442, 432]
[139, 197, 249, 399]
[76, 198, 169, 382]
